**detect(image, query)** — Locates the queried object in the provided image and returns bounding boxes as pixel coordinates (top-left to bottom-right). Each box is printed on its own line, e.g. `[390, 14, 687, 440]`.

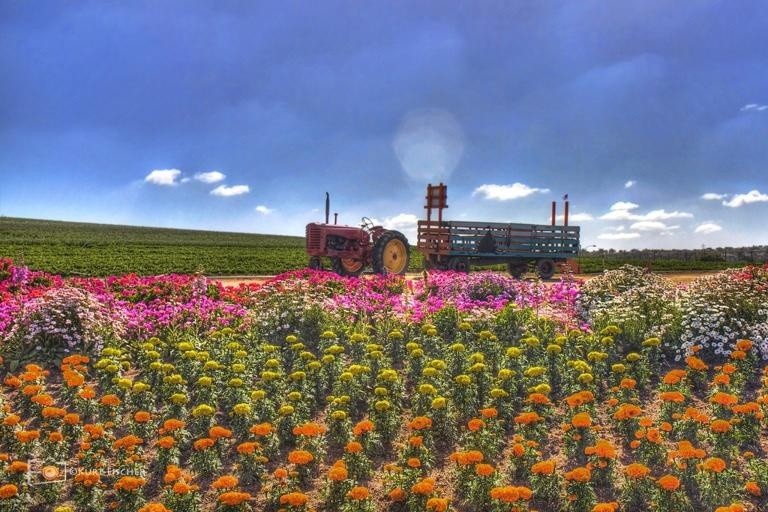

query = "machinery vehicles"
[306, 182, 581, 277]
